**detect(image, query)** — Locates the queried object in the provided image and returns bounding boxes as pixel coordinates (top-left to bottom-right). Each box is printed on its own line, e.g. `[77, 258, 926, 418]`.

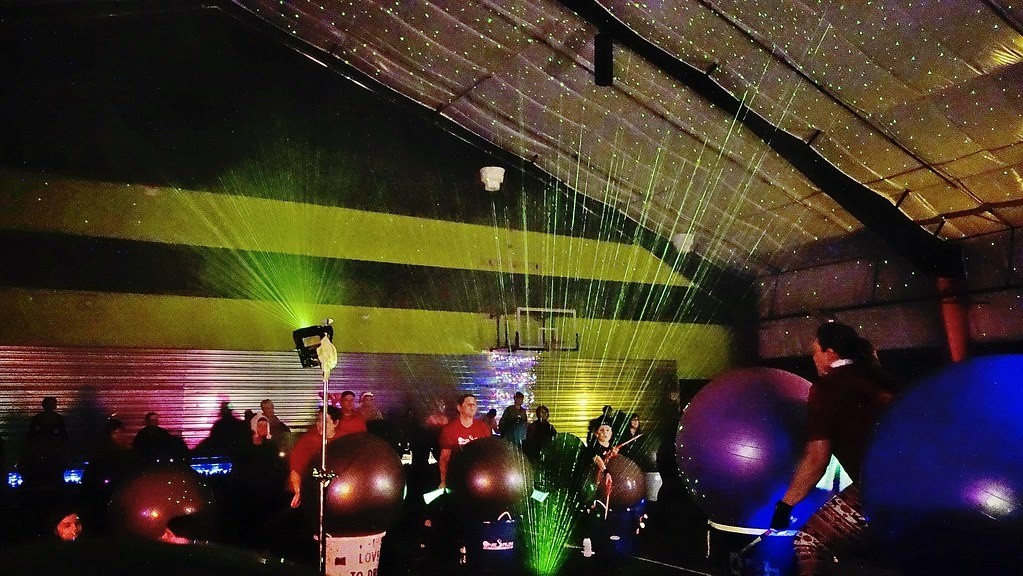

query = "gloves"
[770, 500, 792, 532]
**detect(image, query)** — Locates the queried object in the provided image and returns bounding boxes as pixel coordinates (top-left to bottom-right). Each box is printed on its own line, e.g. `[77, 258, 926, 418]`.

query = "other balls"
[116, 469, 215, 545]
[674, 367, 833, 528]
[861, 356, 1022, 537]
[449, 437, 535, 519]
[304, 431, 408, 536]
[596, 454, 646, 504]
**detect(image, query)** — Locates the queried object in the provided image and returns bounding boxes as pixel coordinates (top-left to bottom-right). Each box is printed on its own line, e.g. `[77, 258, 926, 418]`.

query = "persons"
[161, 514, 196, 544]
[766, 321, 903, 533]
[26, 391, 648, 505]
[47, 508, 84, 541]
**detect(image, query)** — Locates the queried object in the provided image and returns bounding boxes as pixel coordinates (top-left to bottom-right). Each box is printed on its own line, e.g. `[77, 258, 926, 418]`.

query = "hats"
[595, 415, 612, 433]
[251, 411, 272, 440]
[47, 504, 78, 532]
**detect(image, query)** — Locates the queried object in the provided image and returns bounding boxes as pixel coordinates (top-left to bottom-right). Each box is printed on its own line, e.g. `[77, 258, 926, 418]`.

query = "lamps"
[293, 323, 334, 370]
